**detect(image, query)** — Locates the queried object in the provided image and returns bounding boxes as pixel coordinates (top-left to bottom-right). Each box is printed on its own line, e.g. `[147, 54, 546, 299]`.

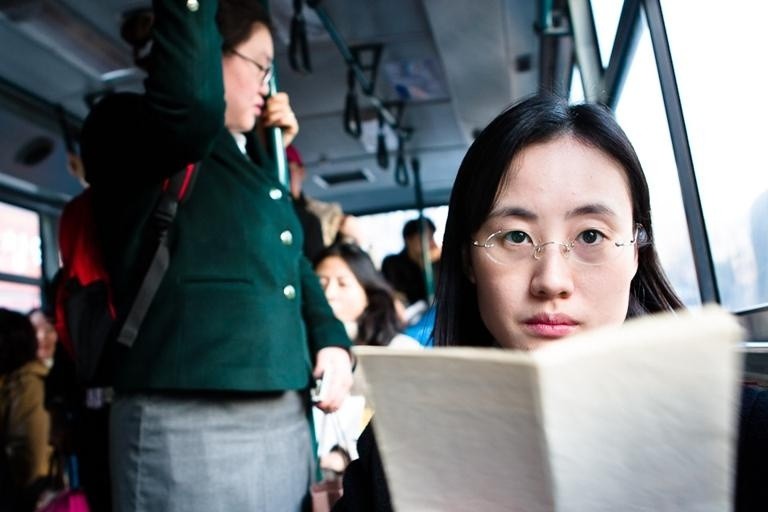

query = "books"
[344, 292, 746, 512]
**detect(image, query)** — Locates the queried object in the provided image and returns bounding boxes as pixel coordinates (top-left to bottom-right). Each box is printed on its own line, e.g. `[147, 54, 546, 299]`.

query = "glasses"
[227, 44, 274, 87]
[473, 223, 642, 267]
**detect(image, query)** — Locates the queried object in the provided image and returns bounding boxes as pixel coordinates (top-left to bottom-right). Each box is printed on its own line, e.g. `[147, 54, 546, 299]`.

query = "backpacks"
[41, 91, 143, 372]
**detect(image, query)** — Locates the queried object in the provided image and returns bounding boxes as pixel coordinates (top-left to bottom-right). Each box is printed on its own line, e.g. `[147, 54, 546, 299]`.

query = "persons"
[280, 137, 333, 264]
[97, 0, 358, 511]
[378, 215, 448, 311]
[50, 81, 152, 512]
[311, 242, 431, 511]
[320, 90, 765, 512]
[0, 306, 57, 512]
[26, 308, 81, 454]
